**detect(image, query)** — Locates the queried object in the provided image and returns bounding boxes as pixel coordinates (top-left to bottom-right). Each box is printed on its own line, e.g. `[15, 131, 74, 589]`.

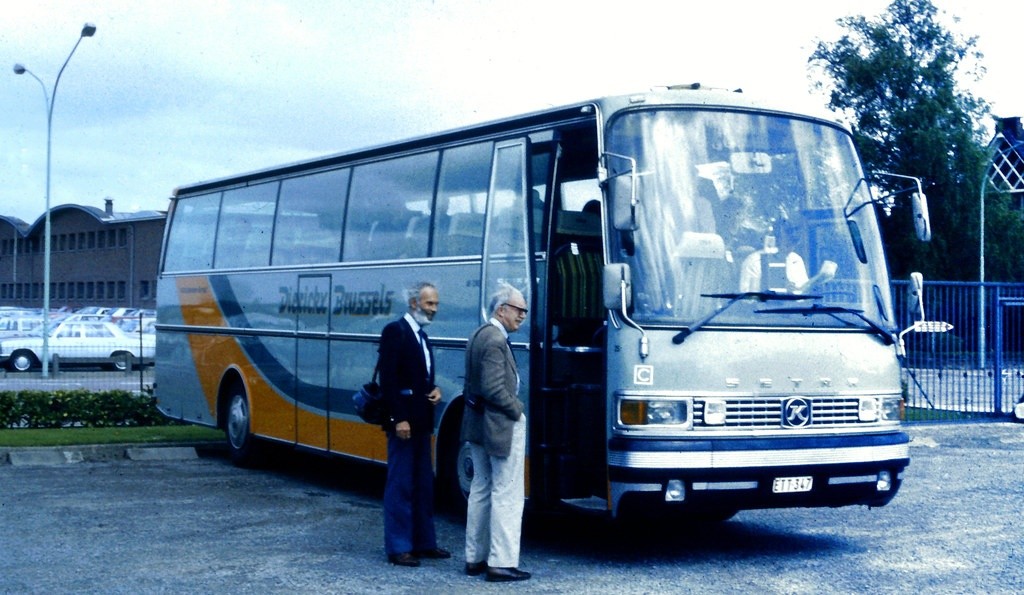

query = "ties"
[507, 336, 517, 363]
[417, 329, 424, 346]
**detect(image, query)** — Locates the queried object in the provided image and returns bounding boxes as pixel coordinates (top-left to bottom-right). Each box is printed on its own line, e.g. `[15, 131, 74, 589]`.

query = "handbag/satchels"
[352, 383, 384, 425]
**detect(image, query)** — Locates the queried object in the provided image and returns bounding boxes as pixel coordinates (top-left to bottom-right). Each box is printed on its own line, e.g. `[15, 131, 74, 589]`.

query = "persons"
[715, 194, 764, 252]
[464, 281, 532, 583]
[382, 195, 451, 238]
[738, 213, 812, 303]
[378, 282, 452, 568]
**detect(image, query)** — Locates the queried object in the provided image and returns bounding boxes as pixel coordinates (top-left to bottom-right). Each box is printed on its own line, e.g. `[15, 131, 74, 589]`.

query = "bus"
[152, 79, 934, 531]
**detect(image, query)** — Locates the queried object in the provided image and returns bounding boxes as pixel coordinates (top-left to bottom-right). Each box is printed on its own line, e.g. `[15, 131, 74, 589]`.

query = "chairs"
[181, 211, 602, 325]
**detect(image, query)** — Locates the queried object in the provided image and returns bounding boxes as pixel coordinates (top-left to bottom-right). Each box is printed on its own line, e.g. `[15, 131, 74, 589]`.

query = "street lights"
[12, 21, 98, 379]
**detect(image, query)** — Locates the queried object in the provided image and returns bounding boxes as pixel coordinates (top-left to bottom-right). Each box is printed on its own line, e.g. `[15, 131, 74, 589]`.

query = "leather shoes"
[388, 552, 420, 566]
[466, 561, 487, 576]
[412, 548, 450, 558]
[485, 567, 531, 582]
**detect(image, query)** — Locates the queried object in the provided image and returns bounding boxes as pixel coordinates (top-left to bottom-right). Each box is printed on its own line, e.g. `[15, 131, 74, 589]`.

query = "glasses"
[500, 303, 528, 315]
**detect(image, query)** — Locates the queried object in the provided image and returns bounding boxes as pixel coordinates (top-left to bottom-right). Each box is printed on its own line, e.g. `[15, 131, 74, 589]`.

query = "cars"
[0, 303, 157, 372]
